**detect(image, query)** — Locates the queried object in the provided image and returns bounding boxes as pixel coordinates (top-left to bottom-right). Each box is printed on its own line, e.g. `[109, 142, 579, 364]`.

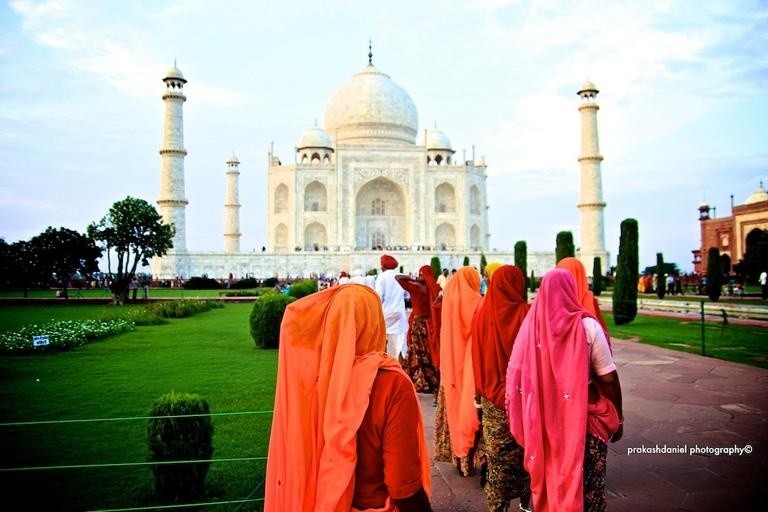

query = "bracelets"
[617, 418, 626, 429]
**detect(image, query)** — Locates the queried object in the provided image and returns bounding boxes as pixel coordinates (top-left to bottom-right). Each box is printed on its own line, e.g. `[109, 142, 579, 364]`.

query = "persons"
[263, 285, 433, 512]
[226, 273, 233, 288]
[505, 269, 624, 512]
[638, 272, 743, 298]
[432, 266, 484, 478]
[474, 266, 532, 512]
[556, 257, 611, 355]
[441, 269, 451, 291]
[757, 269, 767, 297]
[395, 266, 443, 392]
[131, 277, 140, 300]
[485, 262, 504, 293]
[55, 288, 64, 297]
[317, 270, 375, 290]
[375, 256, 410, 368]
[436, 267, 448, 285]
[275, 281, 291, 294]
